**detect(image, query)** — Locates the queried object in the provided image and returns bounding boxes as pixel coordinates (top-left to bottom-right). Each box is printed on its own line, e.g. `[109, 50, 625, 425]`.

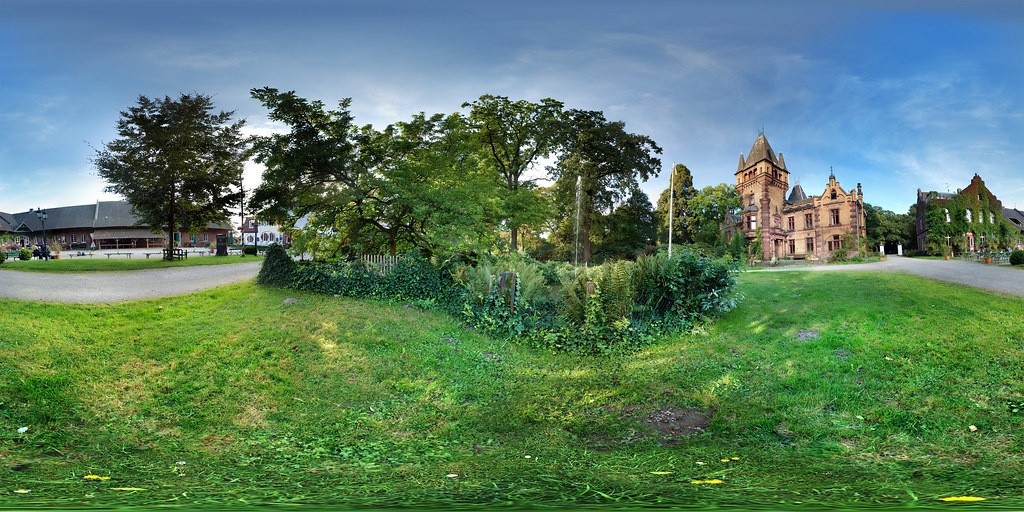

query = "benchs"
[67, 247, 243, 262]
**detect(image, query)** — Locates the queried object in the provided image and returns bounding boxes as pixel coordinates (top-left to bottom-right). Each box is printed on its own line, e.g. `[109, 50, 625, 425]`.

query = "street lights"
[35, 206, 48, 261]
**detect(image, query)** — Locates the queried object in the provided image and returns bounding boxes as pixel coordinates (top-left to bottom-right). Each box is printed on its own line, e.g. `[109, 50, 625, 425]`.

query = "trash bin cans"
[215, 234, 228, 256]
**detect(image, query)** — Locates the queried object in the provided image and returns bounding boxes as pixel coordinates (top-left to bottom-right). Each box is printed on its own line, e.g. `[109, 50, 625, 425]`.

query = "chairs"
[14, 252, 33, 261]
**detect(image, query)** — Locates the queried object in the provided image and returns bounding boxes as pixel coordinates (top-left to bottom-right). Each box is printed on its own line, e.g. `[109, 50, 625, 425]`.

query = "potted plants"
[944, 249, 951, 260]
[984, 253, 993, 264]
